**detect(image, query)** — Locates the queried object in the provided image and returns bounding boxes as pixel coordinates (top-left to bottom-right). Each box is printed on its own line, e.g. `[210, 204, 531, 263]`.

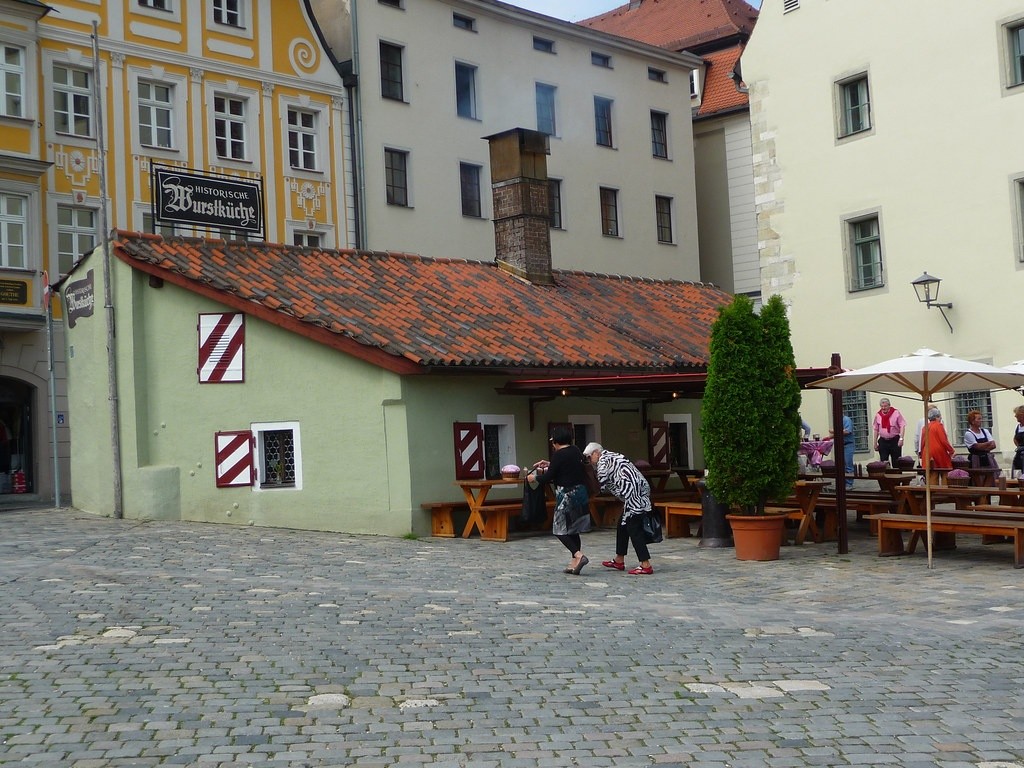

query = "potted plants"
[696, 290, 802, 562]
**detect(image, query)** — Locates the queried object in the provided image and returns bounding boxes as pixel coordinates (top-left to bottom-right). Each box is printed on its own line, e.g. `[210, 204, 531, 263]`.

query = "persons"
[964, 410, 1000, 486]
[527, 429, 592, 574]
[583, 442, 653, 575]
[921, 408, 955, 485]
[799, 416, 811, 442]
[823, 408, 855, 490]
[1011, 405, 1024, 480]
[914, 404, 944, 468]
[872, 398, 906, 468]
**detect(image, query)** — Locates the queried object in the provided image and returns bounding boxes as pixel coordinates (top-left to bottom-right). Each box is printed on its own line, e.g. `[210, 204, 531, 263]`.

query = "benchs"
[421, 496, 602, 542]
[600, 491, 801, 547]
[784, 488, 1024, 568]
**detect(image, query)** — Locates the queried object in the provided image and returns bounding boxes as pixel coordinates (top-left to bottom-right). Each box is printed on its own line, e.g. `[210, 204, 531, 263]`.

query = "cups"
[523, 467, 528, 478]
[991, 496, 999, 506]
[999, 477, 1006, 490]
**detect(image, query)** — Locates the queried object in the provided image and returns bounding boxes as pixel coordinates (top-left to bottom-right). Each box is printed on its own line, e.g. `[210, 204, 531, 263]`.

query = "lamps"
[912, 271, 953, 309]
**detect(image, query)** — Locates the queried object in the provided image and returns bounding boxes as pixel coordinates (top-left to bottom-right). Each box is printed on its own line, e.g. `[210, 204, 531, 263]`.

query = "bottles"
[854, 463, 858, 477]
[858, 463, 862, 477]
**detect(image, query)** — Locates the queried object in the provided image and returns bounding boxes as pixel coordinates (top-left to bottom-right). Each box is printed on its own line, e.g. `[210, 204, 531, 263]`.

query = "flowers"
[948, 469, 969, 480]
[820, 460, 835, 466]
[866, 461, 887, 468]
[898, 455, 914, 461]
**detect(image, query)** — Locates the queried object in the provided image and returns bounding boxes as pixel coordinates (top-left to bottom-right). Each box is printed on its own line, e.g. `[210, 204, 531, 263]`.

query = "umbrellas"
[805, 346, 1024, 568]
[1000, 359, 1024, 396]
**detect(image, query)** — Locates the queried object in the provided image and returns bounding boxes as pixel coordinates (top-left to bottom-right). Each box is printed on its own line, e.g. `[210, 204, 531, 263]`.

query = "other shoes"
[629, 565, 654, 574]
[572, 554, 588, 574]
[563, 566, 572, 572]
[601, 557, 624, 570]
[846, 486, 853, 491]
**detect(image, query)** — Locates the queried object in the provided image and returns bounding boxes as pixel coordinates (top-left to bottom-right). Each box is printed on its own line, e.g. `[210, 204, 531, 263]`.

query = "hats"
[549, 430, 570, 445]
[927, 408, 941, 420]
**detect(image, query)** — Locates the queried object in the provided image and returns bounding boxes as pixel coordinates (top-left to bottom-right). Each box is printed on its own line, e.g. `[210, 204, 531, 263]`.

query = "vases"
[946, 478, 969, 488]
[898, 460, 914, 470]
[952, 455, 970, 461]
[867, 468, 884, 473]
[952, 461, 970, 469]
[821, 466, 835, 474]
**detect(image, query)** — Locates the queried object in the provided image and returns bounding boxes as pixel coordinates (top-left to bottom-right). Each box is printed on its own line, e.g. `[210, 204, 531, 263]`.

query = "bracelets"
[899, 437, 903, 440]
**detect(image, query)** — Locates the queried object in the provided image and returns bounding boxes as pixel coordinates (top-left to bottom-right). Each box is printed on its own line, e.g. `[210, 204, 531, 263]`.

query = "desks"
[453, 477, 554, 540]
[895, 485, 1024, 514]
[784, 482, 831, 547]
[795, 473, 916, 491]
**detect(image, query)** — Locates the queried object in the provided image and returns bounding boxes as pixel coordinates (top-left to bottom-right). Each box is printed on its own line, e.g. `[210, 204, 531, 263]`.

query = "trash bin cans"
[697, 483, 736, 547]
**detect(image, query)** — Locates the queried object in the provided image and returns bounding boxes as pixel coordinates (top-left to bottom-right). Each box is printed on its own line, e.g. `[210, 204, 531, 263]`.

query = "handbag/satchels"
[642, 513, 663, 543]
[523, 469, 547, 522]
[574, 446, 600, 496]
